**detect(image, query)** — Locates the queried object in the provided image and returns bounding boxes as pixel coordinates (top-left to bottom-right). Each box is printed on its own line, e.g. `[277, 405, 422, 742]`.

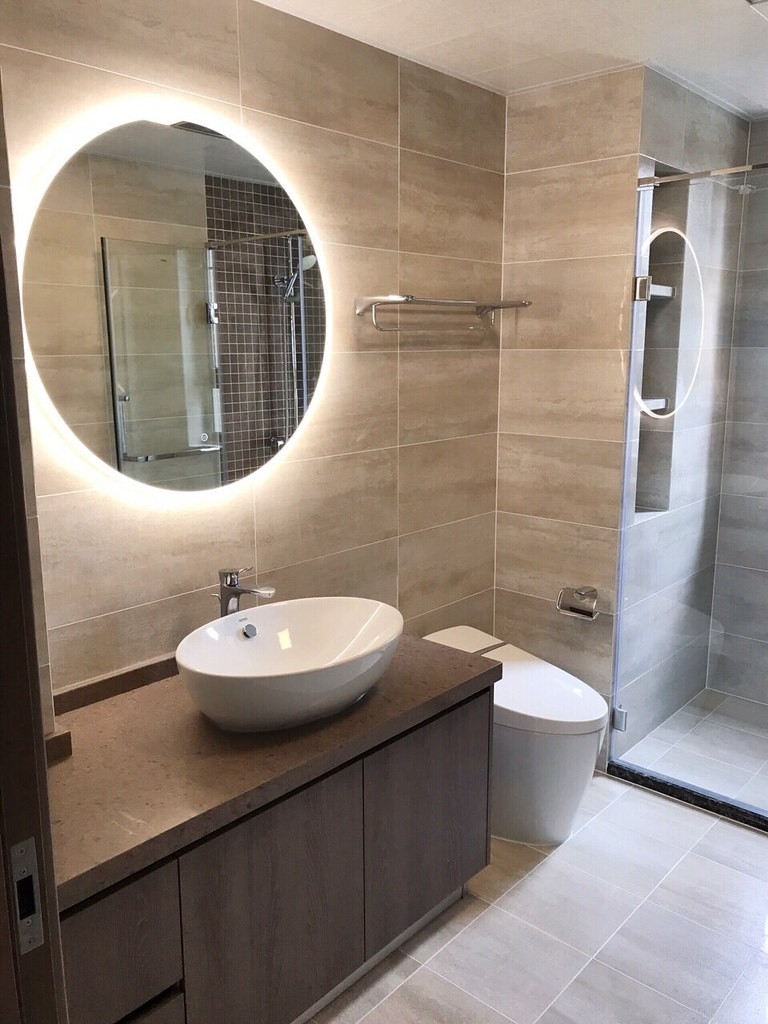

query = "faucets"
[215, 565, 278, 620]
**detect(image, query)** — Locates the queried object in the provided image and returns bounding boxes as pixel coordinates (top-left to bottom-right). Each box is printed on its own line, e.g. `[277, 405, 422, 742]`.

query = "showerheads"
[280, 254, 322, 301]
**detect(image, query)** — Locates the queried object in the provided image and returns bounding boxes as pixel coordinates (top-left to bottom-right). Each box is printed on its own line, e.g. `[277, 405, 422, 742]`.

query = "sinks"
[173, 594, 404, 736]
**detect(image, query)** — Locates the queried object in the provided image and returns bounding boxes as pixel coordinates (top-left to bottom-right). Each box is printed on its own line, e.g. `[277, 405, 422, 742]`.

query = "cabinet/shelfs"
[354, 292, 535, 339]
[56, 622, 505, 1023]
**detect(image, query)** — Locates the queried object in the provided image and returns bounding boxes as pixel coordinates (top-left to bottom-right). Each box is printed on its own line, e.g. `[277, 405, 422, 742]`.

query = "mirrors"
[20, 117, 331, 493]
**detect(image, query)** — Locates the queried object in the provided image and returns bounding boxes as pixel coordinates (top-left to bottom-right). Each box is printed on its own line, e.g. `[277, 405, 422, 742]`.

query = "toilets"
[415, 624, 610, 848]
